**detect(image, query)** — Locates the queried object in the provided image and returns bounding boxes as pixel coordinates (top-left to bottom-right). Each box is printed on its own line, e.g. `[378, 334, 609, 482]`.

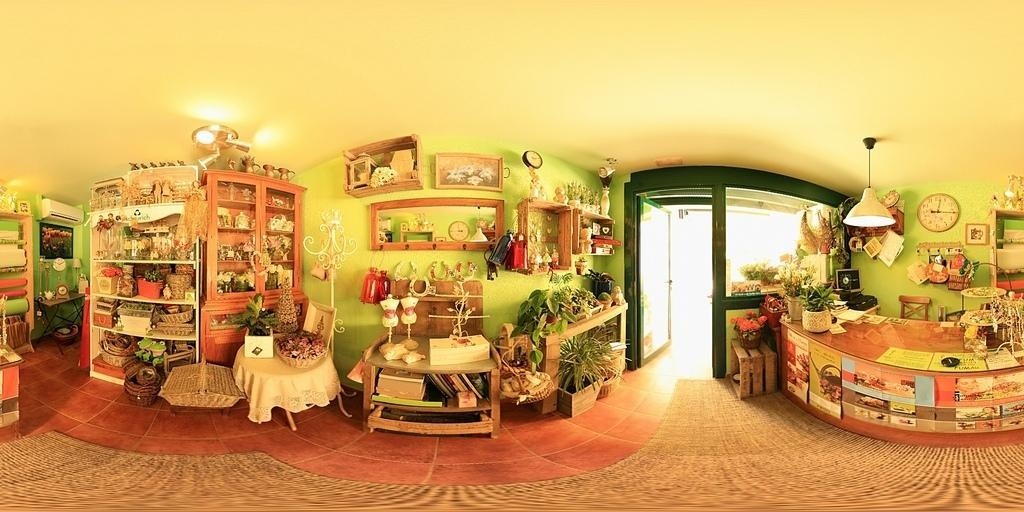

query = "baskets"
[98, 333, 161, 406]
[165, 273, 192, 299]
[599, 368, 621, 398]
[54, 324, 78, 345]
[500, 371, 554, 405]
[274, 339, 328, 369]
[152, 322, 194, 337]
[160, 309, 193, 324]
[157, 354, 248, 408]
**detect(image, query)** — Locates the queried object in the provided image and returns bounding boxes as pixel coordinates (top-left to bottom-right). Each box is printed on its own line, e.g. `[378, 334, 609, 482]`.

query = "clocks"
[916, 192, 960, 232]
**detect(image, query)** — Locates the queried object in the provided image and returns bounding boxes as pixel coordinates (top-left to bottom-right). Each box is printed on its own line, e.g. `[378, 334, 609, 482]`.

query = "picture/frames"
[965, 223, 990, 245]
[39, 222, 73, 259]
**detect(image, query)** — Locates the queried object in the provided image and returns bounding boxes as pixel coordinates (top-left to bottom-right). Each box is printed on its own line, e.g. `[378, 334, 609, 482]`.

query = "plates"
[941, 357, 959, 367]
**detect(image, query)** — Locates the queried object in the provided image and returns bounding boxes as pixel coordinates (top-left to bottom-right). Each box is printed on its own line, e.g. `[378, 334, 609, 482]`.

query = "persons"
[96, 213, 124, 259]
[378, 293, 400, 328]
[401, 291, 420, 326]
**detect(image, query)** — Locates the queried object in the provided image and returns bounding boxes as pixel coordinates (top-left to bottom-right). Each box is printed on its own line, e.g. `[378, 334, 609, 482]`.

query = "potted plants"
[510, 267, 632, 419]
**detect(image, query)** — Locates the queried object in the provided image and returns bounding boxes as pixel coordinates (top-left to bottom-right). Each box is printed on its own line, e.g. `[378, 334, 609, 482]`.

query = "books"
[838, 309, 867, 321]
[371, 369, 489, 422]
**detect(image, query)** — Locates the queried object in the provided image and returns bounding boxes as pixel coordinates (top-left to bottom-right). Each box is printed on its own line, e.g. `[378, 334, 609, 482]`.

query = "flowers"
[42, 227, 72, 256]
[733, 249, 839, 349]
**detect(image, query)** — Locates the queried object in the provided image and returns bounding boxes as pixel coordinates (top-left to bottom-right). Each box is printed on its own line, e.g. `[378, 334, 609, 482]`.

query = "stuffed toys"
[135, 337, 153, 358]
[143, 341, 166, 366]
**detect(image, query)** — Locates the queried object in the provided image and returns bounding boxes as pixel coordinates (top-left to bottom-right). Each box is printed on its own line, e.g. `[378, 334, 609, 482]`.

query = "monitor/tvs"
[836, 269, 860, 292]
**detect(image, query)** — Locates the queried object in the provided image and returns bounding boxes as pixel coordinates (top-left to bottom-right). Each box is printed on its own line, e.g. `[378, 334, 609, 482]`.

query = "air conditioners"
[42, 198, 84, 225]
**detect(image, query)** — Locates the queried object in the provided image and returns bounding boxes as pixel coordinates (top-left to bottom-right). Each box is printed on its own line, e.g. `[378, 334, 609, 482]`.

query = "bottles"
[376, 270, 389, 304]
[488, 228, 515, 265]
[511, 233, 526, 270]
[359, 267, 378, 303]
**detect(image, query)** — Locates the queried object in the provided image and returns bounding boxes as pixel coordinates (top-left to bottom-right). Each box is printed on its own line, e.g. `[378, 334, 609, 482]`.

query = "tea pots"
[240, 160, 296, 182]
[235, 211, 250, 229]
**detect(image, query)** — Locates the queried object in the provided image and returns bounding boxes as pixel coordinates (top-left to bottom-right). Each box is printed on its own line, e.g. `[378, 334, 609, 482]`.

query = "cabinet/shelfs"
[989, 207, 1024, 296]
[0, 211, 34, 355]
[517, 197, 573, 275]
[573, 208, 614, 256]
[87, 134, 505, 439]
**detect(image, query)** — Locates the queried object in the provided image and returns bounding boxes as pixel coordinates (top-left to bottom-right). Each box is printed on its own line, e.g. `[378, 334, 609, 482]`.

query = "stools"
[899, 296, 930, 321]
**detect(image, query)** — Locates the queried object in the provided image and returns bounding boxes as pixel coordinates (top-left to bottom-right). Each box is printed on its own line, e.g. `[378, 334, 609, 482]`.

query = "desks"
[34, 290, 85, 355]
[0, 344, 26, 428]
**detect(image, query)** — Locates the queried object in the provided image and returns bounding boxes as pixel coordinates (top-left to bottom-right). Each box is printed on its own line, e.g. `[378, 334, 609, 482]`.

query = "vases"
[600, 189, 610, 216]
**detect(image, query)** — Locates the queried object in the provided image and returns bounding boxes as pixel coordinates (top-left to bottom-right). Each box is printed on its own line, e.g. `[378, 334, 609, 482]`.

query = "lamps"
[73, 258, 81, 293]
[598, 157, 616, 178]
[843, 138, 896, 226]
[1005, 175, 1018, 197]
[191, 125, 251, 170]
